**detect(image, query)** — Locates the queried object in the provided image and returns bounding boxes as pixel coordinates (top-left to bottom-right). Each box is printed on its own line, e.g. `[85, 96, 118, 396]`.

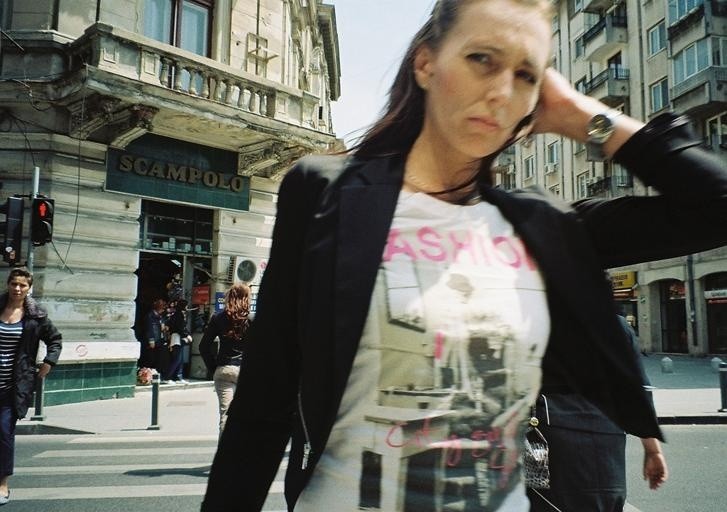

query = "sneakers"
[0, 489, 10, 504]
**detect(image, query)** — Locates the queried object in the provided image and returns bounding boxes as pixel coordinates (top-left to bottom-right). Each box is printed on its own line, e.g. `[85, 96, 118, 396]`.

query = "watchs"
[585, 106, 621, 162]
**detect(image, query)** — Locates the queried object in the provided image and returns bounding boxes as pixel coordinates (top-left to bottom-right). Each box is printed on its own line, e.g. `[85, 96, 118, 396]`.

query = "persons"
[1, 267, 62, 505]
[200, 283, 253, 446]
[143, 299, 165, 367]
[162, 299, 193, 385]
[199, 1, 727, 512]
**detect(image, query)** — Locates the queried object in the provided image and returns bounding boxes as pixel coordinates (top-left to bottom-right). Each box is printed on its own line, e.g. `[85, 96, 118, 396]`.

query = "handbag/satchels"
[523, 403, 550, 490]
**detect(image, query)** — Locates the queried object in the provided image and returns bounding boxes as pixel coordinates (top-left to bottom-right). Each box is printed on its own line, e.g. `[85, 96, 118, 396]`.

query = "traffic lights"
[0, 197, 23, 265]
[30, 196, 55, 246]
